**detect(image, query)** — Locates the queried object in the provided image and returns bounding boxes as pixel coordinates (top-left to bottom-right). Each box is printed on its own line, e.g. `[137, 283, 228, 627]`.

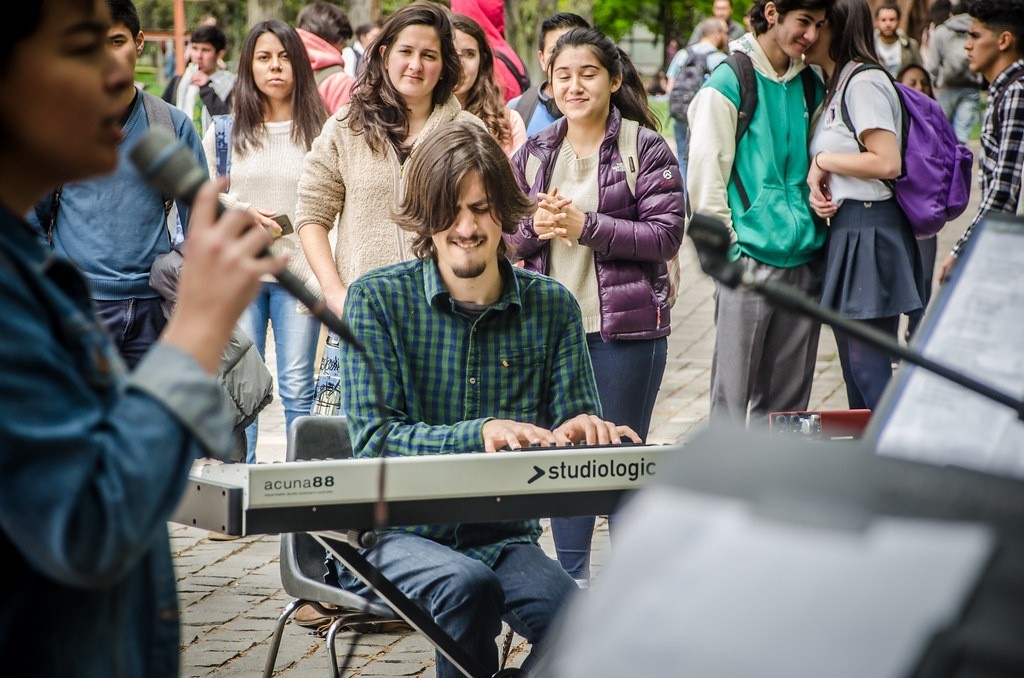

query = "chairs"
[263, 415, 411, 678]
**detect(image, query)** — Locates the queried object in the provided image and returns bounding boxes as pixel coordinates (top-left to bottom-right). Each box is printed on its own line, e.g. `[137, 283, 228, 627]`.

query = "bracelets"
[815, 151, 823, 171]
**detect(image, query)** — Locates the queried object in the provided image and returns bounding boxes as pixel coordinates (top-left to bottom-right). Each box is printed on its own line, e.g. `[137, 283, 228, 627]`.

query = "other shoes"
[293, 600, 336, 625]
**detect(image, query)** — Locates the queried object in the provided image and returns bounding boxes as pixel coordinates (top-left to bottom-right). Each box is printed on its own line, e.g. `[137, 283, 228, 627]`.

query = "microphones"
[128, 125, 364, 352]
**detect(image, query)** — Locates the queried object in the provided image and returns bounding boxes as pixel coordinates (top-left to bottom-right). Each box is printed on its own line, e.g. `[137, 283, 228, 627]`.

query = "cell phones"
[261, 213, 294, 240]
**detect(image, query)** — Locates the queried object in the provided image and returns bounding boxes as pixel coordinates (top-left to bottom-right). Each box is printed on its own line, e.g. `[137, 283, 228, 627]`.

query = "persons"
[0, 0, 291, 678]
[336, 118, 647, 678]
[104, 0, 1024, 622]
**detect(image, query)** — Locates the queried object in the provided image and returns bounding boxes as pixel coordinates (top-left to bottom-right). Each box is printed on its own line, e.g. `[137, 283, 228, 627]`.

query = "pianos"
[170, 433, 684, 677]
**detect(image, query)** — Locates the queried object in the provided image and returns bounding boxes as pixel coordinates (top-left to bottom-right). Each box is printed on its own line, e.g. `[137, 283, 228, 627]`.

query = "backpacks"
[670, 47, 727, 124]
[841, 64, 974, 240]
